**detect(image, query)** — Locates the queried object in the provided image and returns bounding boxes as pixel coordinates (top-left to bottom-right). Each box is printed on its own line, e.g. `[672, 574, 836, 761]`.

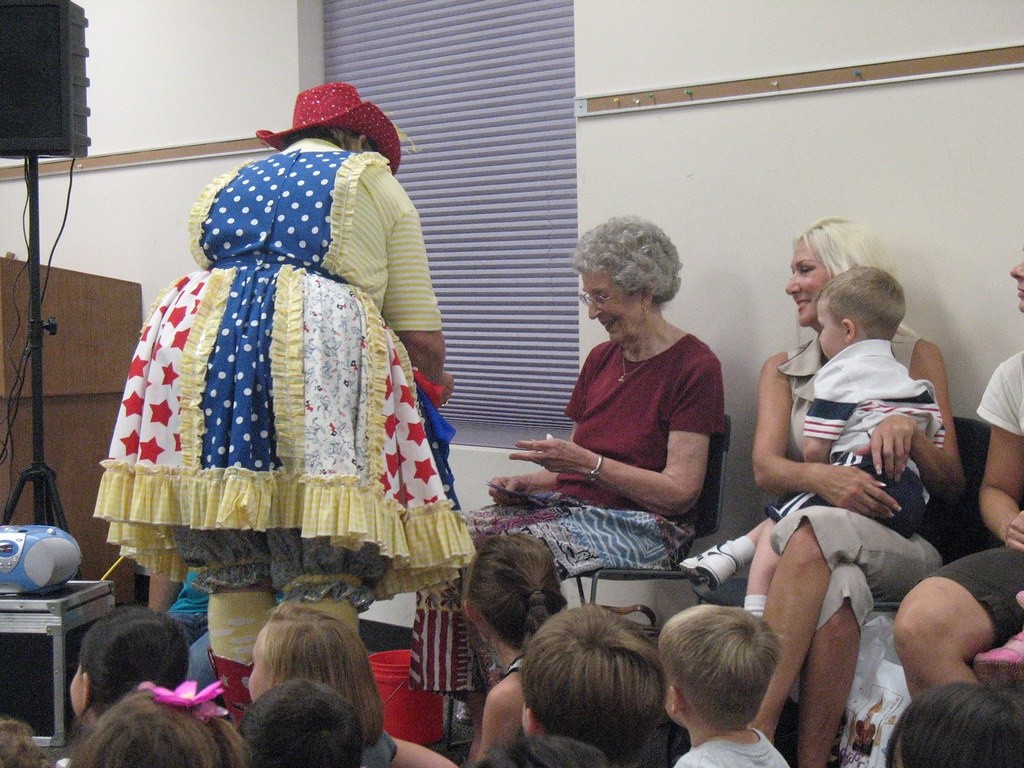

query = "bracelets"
[589, 454, 603, 482]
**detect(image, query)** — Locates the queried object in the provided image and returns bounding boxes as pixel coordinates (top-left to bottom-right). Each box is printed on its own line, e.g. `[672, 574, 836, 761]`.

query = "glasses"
[580, 286, 622, 307]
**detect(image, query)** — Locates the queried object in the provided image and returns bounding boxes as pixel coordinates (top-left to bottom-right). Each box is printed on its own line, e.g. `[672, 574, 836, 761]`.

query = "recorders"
[0, 525, 82, 594]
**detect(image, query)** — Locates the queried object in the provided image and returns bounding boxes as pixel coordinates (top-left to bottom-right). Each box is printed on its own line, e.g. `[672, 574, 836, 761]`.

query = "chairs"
[574, 414, 732, 603]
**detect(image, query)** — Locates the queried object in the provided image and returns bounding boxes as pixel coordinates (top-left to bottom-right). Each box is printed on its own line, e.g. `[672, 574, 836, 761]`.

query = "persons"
[408, 215, 728, 725]
[678, 267, 947, 620]
[92, 82, 477, 732]
[0, 534, 788, 768]
[884, 682, 1024, 768]
[894, 252, 1024, 702]
[746, 216, 962, 768]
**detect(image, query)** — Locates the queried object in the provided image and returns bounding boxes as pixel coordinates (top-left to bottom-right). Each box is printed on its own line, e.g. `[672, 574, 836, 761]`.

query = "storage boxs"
[0, 580, 115, 748]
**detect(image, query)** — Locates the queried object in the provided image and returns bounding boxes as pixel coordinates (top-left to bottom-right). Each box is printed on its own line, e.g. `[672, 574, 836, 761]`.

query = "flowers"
[138, 678, 230, 723]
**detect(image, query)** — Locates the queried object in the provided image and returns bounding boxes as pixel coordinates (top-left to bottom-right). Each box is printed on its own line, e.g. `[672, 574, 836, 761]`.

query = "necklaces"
[618, 321, 667, 382]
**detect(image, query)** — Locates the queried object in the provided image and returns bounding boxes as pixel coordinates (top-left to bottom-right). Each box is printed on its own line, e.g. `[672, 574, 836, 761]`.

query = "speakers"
[0, 0, 91, 159]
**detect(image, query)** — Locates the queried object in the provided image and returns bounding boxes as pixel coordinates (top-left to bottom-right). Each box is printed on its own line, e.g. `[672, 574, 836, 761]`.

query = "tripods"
[0, 155, 70, 533]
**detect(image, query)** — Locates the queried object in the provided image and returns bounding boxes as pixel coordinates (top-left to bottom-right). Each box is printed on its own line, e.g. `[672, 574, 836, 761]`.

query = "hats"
[255, 82, 401, 174]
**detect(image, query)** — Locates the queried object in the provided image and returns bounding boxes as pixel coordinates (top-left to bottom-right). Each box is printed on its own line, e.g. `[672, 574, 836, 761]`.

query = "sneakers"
[678, 539, 740, 592]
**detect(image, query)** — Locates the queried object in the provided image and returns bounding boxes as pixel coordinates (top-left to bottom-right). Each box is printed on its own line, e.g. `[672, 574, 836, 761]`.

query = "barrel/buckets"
[368, 649, 443, 747]
